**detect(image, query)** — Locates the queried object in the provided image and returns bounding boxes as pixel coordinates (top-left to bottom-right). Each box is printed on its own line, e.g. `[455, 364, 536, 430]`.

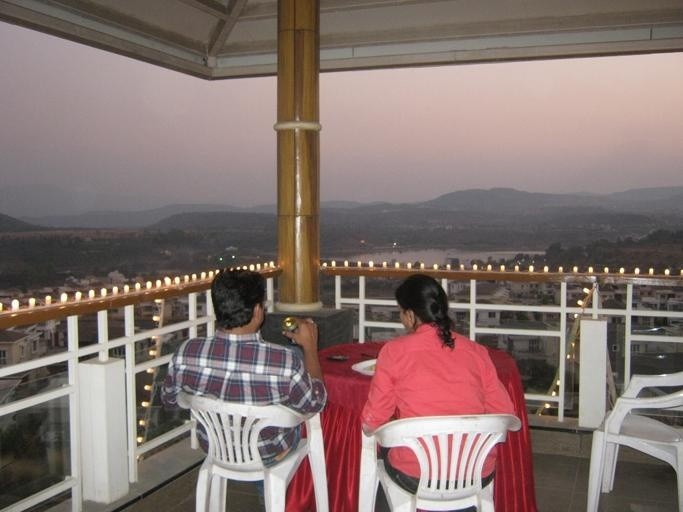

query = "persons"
[160, 271, 327, 496]
[362, 274, 515, 512]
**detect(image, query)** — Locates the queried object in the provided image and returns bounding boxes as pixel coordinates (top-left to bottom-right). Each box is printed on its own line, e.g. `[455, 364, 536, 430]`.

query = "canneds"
[283, 316, 299, 345]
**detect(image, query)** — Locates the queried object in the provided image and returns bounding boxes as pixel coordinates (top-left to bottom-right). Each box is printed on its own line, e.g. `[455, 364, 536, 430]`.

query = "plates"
[351, 359, 377, 376]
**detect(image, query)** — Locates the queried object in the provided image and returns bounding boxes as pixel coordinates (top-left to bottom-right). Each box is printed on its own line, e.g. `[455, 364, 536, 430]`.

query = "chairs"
[176, 391, 334, 511]
[586, 372, 683, 511]
[357, 408, 521, 511]
